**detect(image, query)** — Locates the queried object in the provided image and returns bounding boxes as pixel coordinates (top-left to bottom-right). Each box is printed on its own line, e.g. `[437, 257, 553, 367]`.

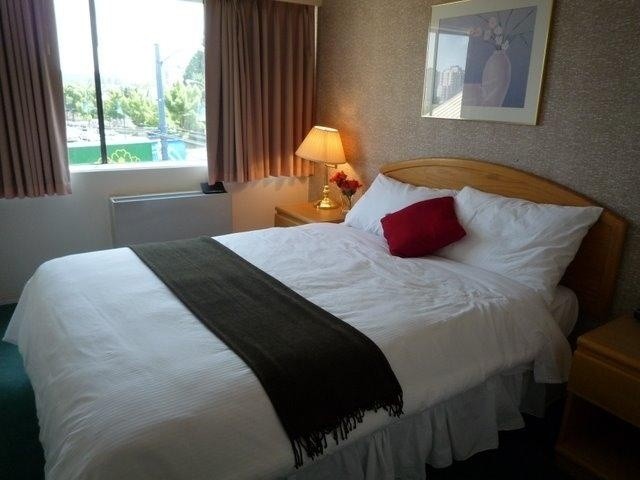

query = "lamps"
[295, 126, 346, 209]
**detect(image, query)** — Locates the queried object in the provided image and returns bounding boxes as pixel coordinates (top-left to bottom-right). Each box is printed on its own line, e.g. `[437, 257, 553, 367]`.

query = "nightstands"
[551, 313, 639, 480]
[274, 200, 348, 227]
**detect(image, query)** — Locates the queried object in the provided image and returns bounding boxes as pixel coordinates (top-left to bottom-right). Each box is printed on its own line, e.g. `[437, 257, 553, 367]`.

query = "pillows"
[341, 172, 604, 305]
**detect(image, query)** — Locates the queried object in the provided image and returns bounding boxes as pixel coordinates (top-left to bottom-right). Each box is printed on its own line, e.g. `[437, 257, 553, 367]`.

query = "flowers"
[330, 170, 362, 210]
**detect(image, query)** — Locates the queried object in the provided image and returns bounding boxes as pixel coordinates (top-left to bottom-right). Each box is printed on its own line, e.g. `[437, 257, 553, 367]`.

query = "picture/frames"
[420, 0, 555, 127]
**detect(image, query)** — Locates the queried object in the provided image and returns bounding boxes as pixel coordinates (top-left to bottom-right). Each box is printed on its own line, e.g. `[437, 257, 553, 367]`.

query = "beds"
[18, 155, 628, 480]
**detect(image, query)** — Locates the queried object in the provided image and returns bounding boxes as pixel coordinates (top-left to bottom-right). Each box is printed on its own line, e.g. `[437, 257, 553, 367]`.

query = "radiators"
[111, 191, 234, 248]
